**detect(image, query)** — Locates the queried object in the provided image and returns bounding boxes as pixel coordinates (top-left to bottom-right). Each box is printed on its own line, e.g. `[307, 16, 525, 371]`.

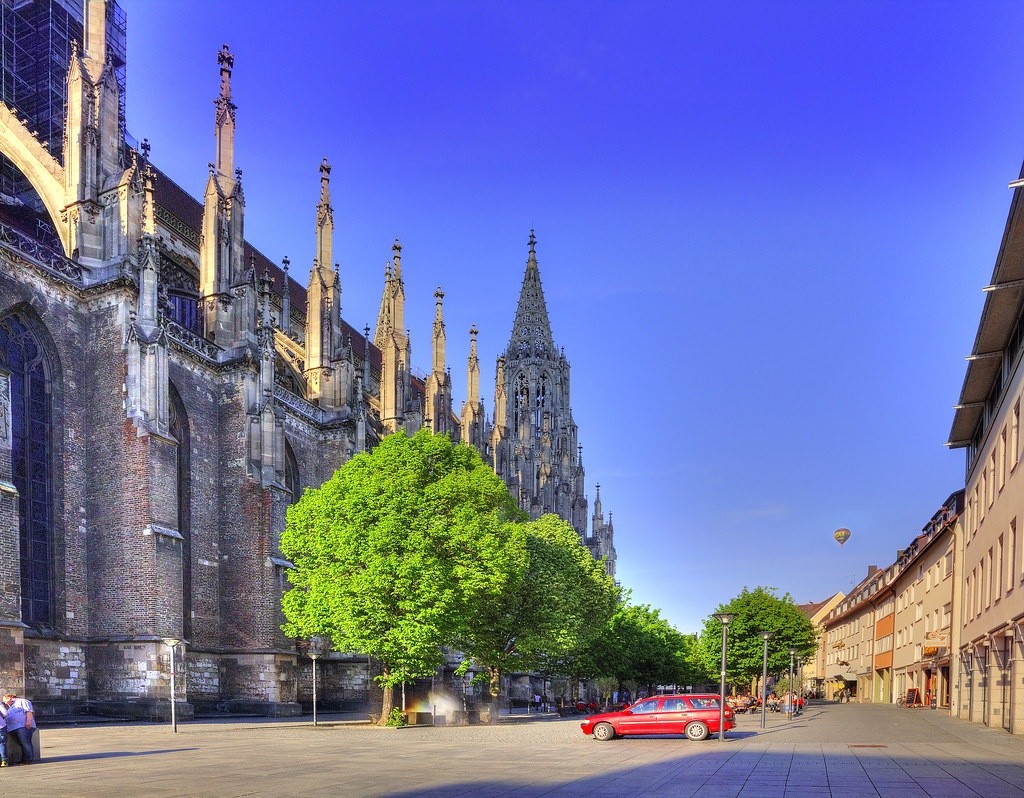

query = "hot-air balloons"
[833, 528, 851, 545]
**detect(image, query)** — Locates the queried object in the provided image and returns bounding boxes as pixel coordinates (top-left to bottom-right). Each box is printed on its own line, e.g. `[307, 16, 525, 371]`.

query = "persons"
[724, 688, 851, 719]
[3, 694, 37, 766]
[0, 699, 10, 767]
[531, 693, 642, 715]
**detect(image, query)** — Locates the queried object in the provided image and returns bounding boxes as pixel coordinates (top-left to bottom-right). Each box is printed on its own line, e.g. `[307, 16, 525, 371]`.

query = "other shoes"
[1, 761, 9, 767]
[18, 760, 34, 764]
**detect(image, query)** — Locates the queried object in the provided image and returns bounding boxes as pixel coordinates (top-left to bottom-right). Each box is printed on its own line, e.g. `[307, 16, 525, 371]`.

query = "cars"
[580, 693, 737, 741]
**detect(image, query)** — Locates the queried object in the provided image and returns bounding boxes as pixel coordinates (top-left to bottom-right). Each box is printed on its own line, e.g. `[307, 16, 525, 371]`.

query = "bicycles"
[896, 691, 905, 708]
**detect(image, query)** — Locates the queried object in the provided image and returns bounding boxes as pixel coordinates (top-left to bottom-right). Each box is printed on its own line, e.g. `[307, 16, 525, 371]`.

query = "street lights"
[799, 659, 806, 699]
[161, 637, 182, 733]
[307, 653, 324, 727]
[711, 613, 740, 741]
[757, 631, 774, 730]
[794, 654, 803, 715]
[786, 648, 799, 722]
